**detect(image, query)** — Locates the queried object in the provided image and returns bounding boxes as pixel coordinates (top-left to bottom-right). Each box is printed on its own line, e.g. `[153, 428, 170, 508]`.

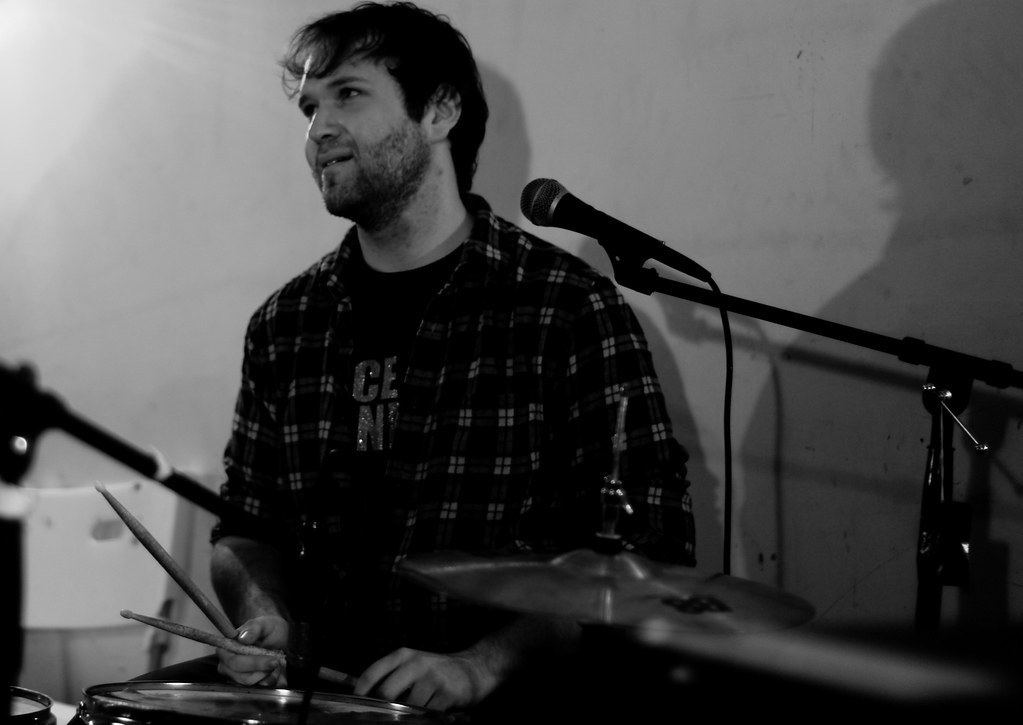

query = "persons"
[208, 1, 697, 716]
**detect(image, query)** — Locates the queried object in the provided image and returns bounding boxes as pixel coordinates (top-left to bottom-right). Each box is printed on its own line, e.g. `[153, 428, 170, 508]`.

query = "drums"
[0, 681, 54, 725]
[74, 680, 420, 725]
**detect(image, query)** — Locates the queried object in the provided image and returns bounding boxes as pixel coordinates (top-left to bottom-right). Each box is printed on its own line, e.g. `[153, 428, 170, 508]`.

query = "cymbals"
[394, 539, 819, 634]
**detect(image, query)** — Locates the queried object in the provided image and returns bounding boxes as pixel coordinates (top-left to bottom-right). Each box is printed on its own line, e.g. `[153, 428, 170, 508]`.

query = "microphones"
[519, 177, 712, 285]
